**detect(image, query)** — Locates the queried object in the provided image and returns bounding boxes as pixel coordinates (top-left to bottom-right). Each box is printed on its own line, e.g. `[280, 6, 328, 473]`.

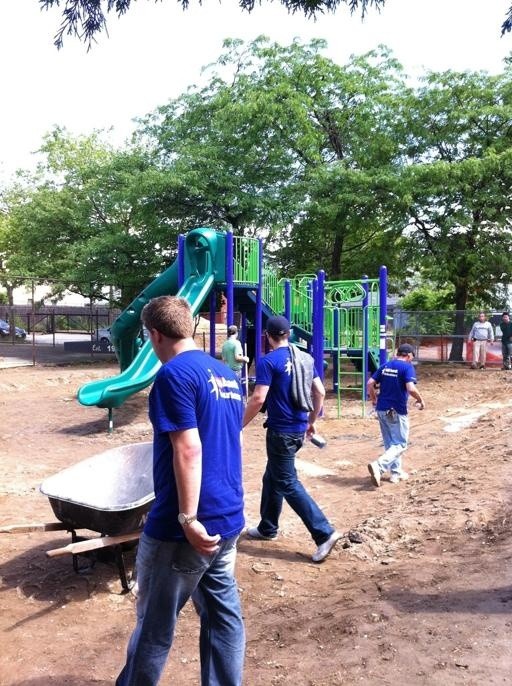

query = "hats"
[398, 343, 415, 356]
[266, 316, 289, 335]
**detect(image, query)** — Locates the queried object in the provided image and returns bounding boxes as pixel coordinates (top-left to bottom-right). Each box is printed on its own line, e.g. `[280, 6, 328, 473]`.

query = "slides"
[79, 227, 226, 408]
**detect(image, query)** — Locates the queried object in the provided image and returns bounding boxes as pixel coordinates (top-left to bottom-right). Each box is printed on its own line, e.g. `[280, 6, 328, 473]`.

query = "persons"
[468, 313, 494, 370]
[500, 312, 512, 368]
[242, 317, 344, 564]
[116, 295, 244, 686]
[221, 325, 249, 382]
[364, 342, 425, 488]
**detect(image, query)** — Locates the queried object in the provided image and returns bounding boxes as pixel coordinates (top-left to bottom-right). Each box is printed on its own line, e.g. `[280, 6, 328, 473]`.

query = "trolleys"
[0, 442, 155, 591]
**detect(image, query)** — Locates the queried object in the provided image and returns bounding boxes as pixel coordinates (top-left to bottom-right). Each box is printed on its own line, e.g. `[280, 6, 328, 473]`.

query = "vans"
[486, 312, 512, 339]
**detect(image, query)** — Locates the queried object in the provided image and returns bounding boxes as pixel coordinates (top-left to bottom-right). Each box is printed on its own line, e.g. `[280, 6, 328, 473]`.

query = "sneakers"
[389, 472, 409, 482]
[247, 527, 276, 540]
[368, 463, 382, 487]
[312, 532, 339, 562]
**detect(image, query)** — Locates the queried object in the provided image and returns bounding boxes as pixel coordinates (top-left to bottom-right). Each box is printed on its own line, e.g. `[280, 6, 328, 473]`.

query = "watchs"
[177, 512, 199, 528]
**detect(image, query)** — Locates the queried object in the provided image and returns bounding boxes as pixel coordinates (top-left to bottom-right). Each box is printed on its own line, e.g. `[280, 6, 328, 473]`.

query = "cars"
[0, 318, 26, 340]
[92, 326, 148, 352]
